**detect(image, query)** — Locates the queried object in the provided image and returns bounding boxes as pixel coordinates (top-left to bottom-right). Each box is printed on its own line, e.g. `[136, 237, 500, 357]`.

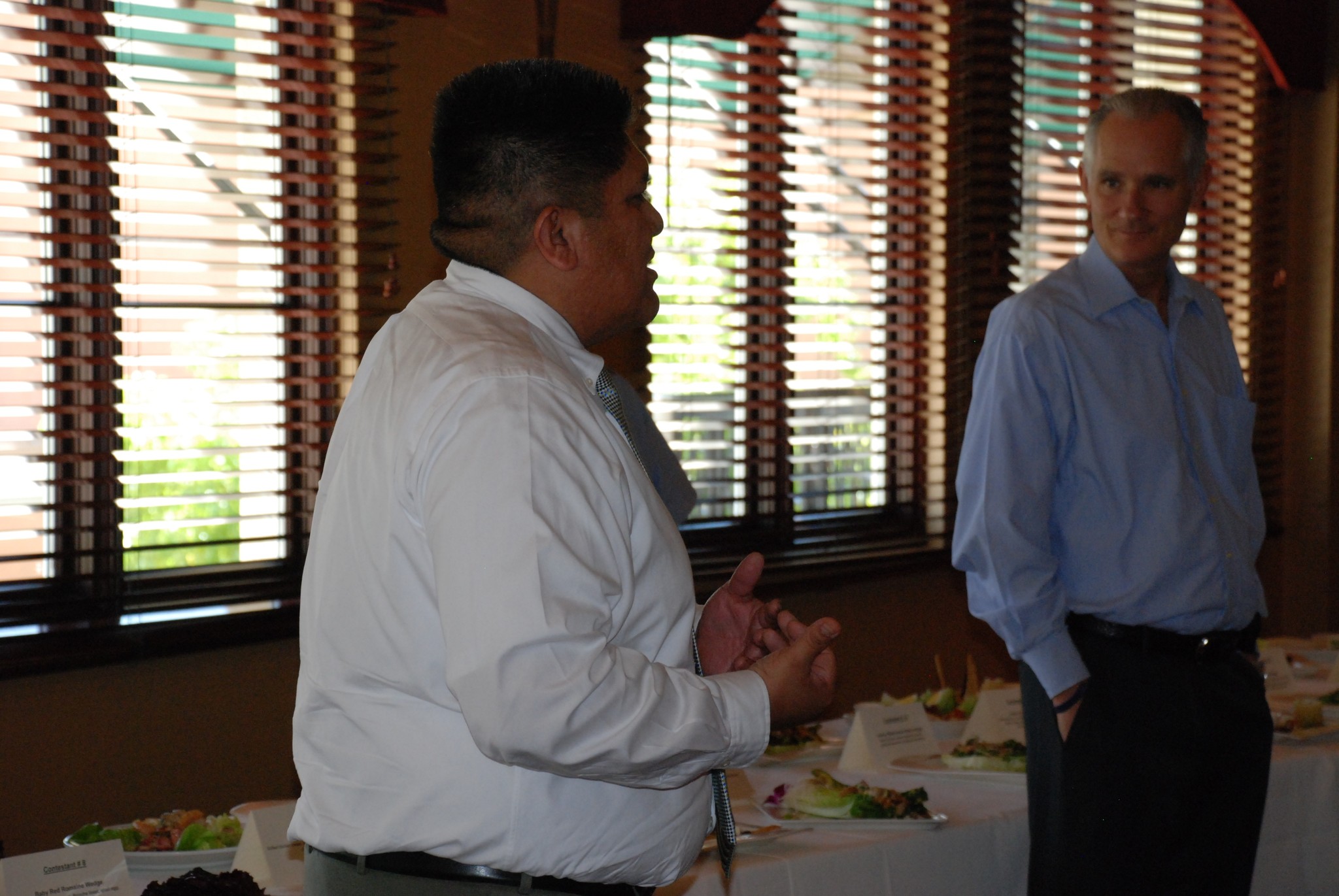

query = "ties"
[596, 370, 650, 477]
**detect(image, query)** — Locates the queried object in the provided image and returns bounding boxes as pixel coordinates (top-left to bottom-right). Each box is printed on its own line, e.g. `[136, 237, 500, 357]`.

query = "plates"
[752, 797, 950, 830]
[887, 752, 1029, 783]
[62, 822, 239, 871]
[229, 802, 247, 824]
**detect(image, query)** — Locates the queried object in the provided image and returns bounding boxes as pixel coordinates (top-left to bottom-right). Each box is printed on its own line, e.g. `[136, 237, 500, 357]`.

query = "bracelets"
[1054, 684, 1085, 713]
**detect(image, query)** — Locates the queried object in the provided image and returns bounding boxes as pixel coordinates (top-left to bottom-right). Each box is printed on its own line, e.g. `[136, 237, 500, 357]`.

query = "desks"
[122, 637, 1339, 896]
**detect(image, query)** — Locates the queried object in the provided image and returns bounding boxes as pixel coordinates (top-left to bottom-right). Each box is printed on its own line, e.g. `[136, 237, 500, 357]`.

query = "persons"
[284, 57, 843, 896]
[950, 87, 1276, 896]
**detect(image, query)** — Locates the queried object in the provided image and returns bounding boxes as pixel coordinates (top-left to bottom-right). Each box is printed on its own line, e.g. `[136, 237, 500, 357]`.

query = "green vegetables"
[69, 813, 241, 852]
[915, 686, 978, 721]
[784, 779, 929, 821]
[953, 737, 1026, 765]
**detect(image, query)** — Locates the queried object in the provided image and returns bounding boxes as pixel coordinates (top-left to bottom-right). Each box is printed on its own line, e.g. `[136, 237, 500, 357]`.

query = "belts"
[334, 847, 655, 896]
